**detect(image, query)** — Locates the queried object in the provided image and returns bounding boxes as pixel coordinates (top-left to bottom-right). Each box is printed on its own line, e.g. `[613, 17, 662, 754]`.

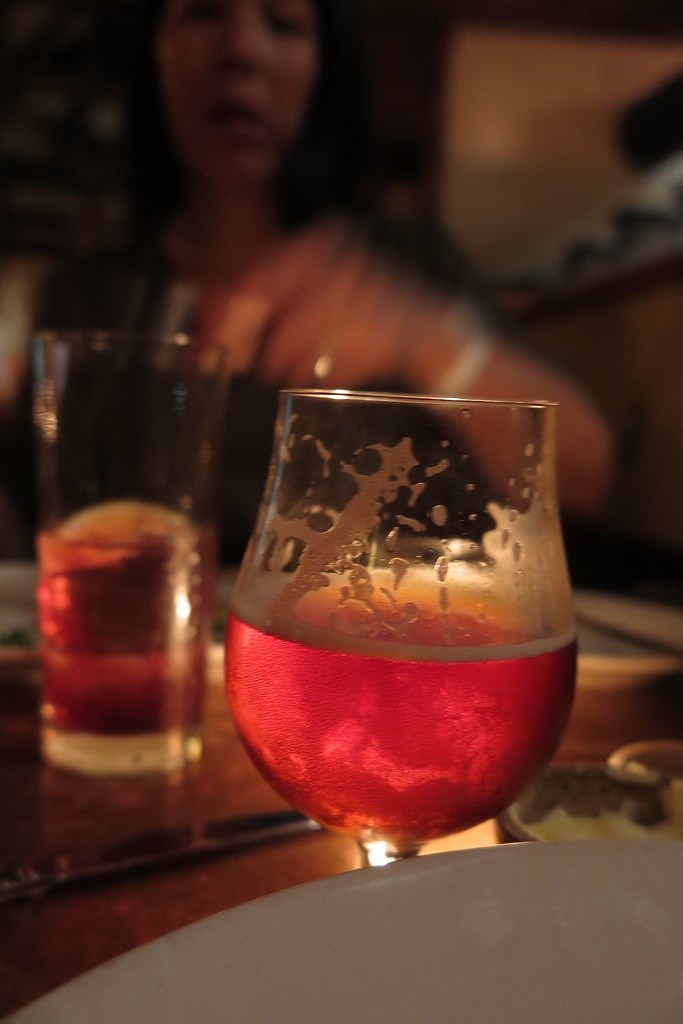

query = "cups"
[37, 325, 230, 775]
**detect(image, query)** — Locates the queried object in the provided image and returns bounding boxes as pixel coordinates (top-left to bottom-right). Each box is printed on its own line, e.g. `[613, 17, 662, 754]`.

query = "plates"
[0, 834, 681, 1024]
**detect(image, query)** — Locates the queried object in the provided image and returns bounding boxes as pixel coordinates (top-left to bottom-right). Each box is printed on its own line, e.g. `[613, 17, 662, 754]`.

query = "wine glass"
[227, 388, 580, 866]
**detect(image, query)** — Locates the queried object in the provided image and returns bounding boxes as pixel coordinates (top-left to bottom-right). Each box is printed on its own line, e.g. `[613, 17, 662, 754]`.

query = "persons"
[0, 0, 612, 560]
